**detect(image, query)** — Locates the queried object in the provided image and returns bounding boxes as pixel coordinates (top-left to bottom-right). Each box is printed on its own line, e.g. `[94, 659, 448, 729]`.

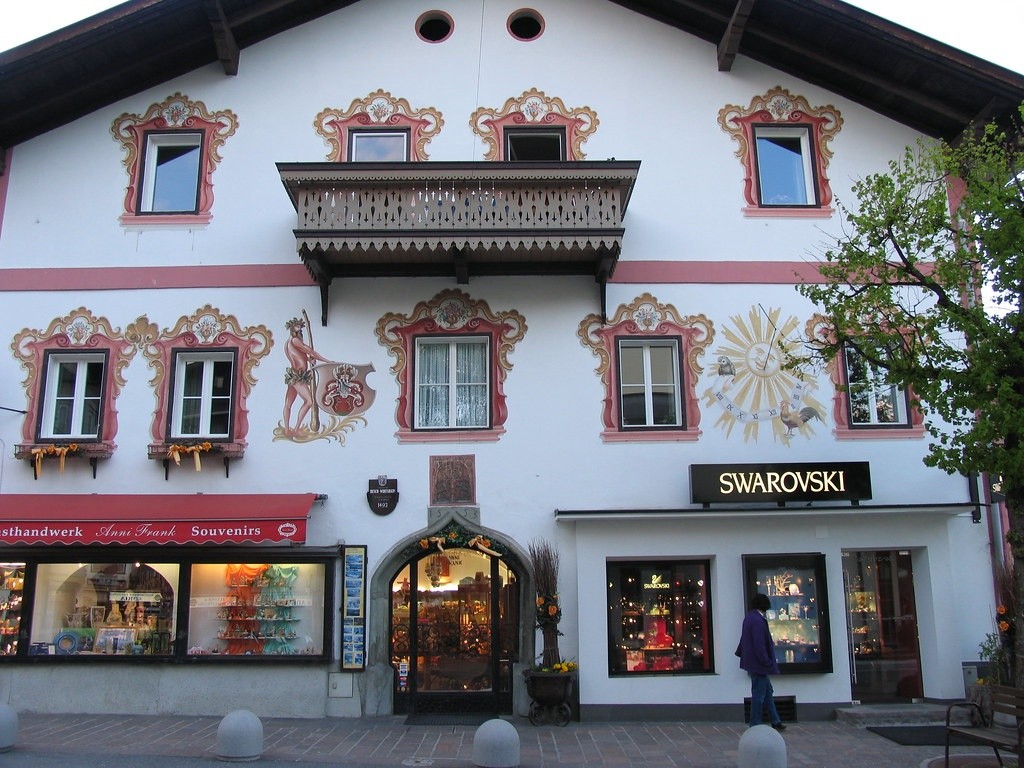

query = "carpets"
[867, 726, 988, 746]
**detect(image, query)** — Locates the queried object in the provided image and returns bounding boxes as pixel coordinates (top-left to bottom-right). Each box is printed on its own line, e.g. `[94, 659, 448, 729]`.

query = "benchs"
[945, 684, 1024, 768]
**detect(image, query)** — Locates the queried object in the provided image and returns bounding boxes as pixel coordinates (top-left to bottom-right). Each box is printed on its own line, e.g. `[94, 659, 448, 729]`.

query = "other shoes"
[772, 722, 786, 730]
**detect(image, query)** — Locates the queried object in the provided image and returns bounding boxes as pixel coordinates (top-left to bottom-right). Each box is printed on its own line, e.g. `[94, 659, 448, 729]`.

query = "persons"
[735, 593, 786, 731]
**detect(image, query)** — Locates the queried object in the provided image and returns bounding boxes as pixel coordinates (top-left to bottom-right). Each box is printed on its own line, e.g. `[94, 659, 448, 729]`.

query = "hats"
[751, 594, 771, 608]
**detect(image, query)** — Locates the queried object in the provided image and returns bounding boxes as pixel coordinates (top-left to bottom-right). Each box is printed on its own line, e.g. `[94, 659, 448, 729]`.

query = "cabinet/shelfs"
[217, 585, 301, 639]
[0, 562, 26, 656]
[765, 563, 883, 663]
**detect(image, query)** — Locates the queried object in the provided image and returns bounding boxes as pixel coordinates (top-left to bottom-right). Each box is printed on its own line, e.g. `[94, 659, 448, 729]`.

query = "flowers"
[978, 543, 1016, 684]
[525, 536, 577, 673]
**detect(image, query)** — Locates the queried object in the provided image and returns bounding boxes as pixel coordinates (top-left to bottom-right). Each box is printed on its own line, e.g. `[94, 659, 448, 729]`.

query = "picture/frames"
[93, 626, 138, 653]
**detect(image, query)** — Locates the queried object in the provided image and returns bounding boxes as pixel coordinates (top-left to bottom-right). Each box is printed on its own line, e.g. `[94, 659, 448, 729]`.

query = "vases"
[523, 670, 577, 727]
[993, 685, 1017, 728]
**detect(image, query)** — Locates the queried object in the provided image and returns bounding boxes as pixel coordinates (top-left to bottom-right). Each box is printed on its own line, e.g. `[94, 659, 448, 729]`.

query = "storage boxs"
[30, 643, 48, 655]
